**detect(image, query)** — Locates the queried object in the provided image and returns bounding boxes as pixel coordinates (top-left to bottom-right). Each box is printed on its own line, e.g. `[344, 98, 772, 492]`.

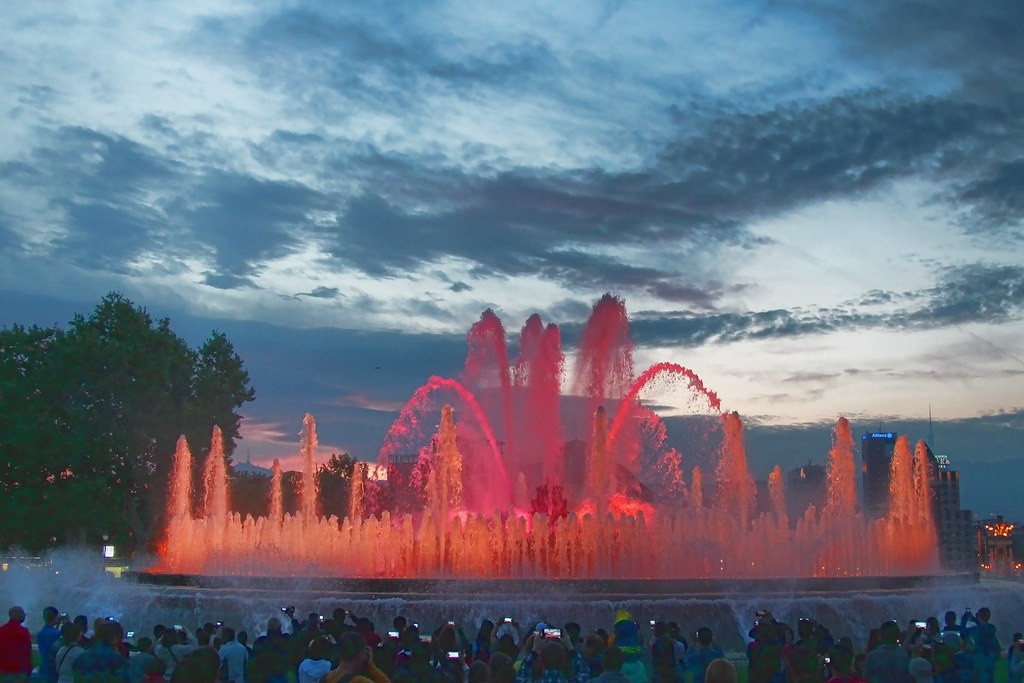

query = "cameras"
[214, 619, 224, 629]
[281, 606, 295, 614]
[57, 612, 69, 624]
[105, 616, 114, 621]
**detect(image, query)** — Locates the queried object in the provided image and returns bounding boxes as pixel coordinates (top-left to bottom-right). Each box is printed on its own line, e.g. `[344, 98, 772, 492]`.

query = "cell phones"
[173, 624, 183, 630]
[126, 631, 135, 638]
[388, 631, 400, 638]
[542, 628, 563, 639]
[417, 634, 433, 643]
[447, 651, 460, 658]
[413, 622, 418, 628]
[503, 617, 513, 624]
[447, 620, 455, 626]
[320, 615, 324, 623]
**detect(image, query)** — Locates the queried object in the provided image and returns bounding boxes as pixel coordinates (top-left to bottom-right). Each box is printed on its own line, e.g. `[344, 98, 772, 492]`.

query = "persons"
[4, 602, 1023, 683]
